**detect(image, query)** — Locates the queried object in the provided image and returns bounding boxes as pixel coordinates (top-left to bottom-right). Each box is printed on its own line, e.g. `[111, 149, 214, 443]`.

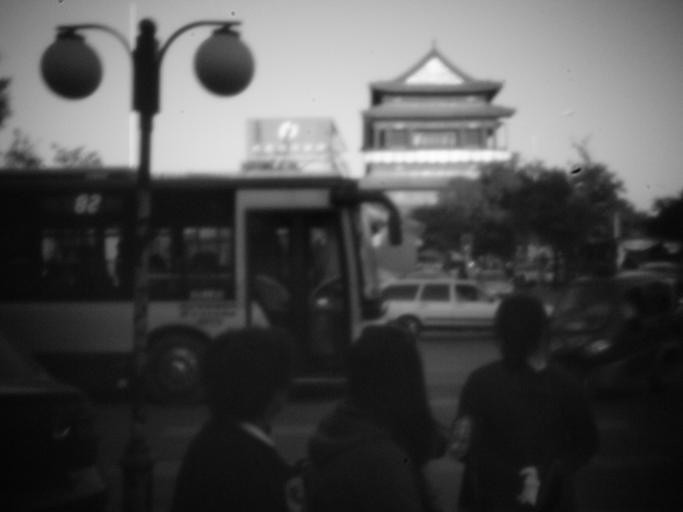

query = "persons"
[163, 323, 291, 510]
[449, 293, 601, 510]
[298, 324, 450, 510]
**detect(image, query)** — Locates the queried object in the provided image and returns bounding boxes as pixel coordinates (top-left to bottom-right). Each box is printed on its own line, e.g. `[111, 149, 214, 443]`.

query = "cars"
[374, 278, 556, 337]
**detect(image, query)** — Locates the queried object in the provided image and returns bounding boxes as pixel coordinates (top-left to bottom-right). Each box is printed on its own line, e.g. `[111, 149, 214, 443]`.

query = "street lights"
[40, 16, 255, 512]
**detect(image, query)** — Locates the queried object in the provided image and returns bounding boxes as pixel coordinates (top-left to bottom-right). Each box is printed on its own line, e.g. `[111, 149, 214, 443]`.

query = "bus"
[0, 167, 402, 400]
